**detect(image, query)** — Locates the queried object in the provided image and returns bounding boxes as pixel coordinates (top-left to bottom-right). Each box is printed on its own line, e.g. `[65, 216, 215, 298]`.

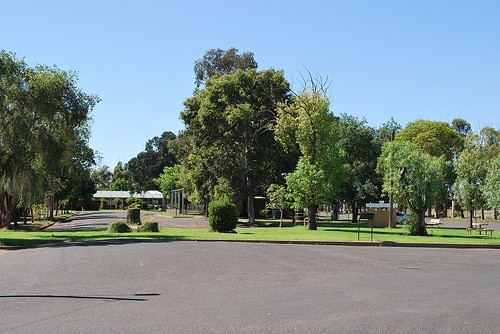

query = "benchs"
[467, 228, 494, 235]
[426, 219, 443, 228]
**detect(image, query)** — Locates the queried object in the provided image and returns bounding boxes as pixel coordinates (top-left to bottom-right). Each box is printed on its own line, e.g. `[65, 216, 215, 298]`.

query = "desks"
[472, 222, 488, 235]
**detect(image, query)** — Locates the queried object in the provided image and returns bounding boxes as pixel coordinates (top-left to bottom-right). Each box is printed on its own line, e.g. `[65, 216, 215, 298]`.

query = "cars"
[396, 211, 413, 225]
